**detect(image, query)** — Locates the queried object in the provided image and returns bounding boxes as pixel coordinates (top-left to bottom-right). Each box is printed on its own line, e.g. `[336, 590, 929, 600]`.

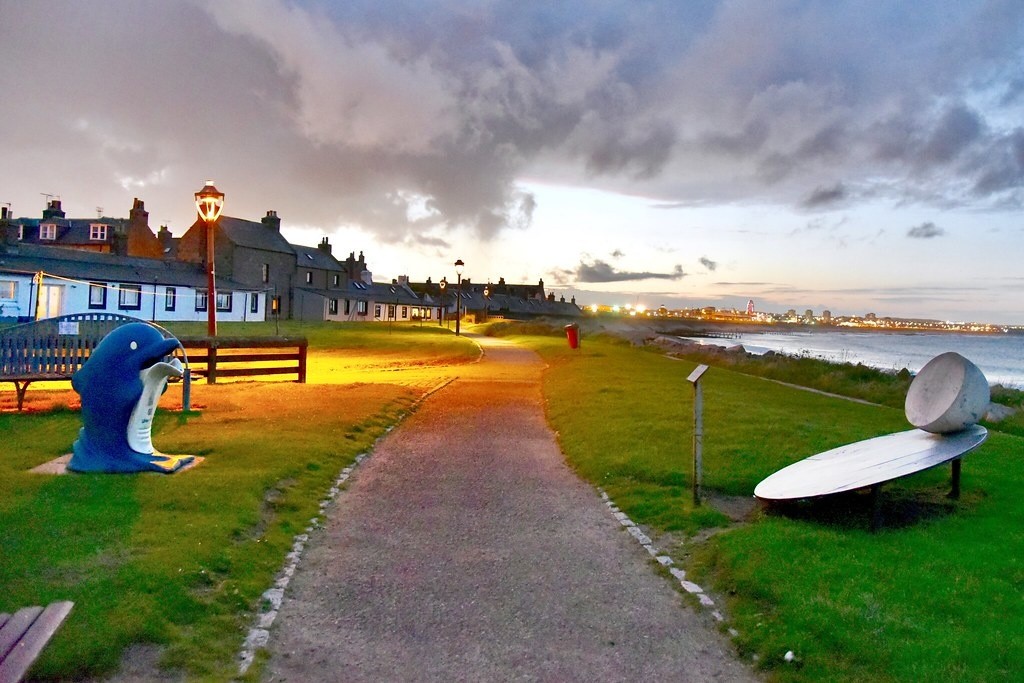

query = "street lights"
[483, 285, 489, 324]
[454, 258, 464, 336]
[193, 178, 226, 385]
[439, 279, 446, 327]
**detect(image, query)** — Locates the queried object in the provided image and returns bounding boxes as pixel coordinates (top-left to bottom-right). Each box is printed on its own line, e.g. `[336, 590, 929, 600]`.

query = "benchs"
[0, 312, 192, 412]
[0, 600, 76, 683]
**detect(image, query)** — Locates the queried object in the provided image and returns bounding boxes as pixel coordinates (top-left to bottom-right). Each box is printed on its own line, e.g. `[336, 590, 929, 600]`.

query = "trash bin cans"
[65, 322, 191, 475]
[564, 323, 580, 349]
